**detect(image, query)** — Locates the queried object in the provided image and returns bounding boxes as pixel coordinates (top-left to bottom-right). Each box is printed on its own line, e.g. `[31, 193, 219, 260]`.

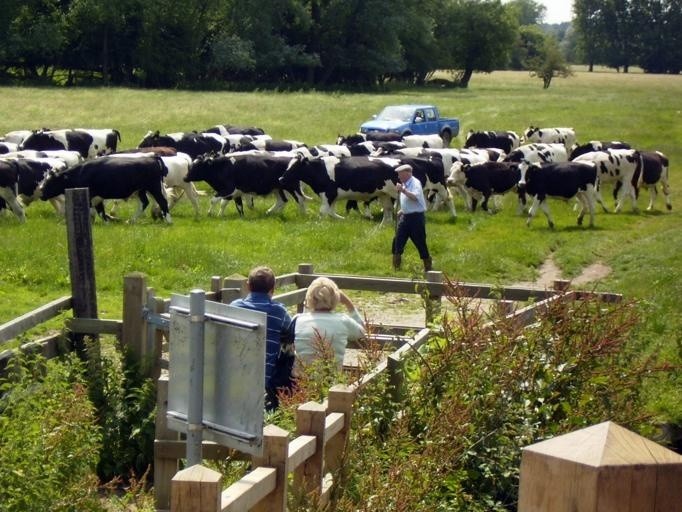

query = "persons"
[290, 276, 366, 390]
[229, 266, 292, 400]
[391, 164, 432, 272]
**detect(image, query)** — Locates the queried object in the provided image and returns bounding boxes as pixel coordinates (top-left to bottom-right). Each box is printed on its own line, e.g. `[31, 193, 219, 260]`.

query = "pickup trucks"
[358, 103, 460, 148]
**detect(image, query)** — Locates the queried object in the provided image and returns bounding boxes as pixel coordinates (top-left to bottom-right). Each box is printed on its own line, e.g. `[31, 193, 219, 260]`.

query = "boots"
[423, 256, 432, 272]
[393, 255, 401, 271]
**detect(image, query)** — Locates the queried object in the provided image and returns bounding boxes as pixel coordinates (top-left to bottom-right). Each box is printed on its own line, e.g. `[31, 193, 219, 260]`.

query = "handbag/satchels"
[276, 339, 293, 374]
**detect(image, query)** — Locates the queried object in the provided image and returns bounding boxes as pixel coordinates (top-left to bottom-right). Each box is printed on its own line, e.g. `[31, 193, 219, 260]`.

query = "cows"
[0, 125, 673, 228]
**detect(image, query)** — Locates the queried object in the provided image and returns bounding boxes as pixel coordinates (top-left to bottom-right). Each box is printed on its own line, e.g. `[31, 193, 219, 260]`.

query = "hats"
[395, 165, 413, 172]
